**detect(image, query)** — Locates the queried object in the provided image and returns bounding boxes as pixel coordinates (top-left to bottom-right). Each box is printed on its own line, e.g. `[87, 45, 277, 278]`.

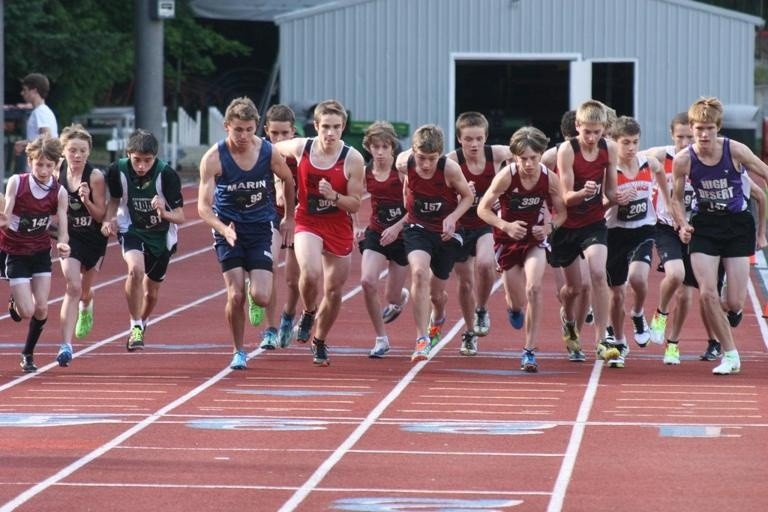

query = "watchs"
[332, 192, 341, 203]
[548, 221, 557, 234]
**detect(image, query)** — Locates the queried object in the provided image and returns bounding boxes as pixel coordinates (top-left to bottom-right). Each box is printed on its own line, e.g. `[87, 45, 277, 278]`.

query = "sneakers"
[711, 357, 741, 375]
[259, 326, 278, 351]
[229, 345, 248, 370]
[606, 343, 629, 369]
[650, 311, 668, 344]
[519, 345, 540, 371]
[730, 310, 742, 327]
[473, 306, 490, 338]
[18, 353, 38, 374]
[275, 308, 296, 348]
[699, 338, 722, 361]
[295, 302, 319, 345]
[366, 336, 391, 358]
[662, 341, 681, 366]
[567, 350, 586, 363]
[126, 325, 147, 349]
[427, 310, 447, 348]
[410, 334, 432, 363]
[460, 330, 479, 357]
[508, 307, 525, 330]
[606, 330, 618, 343]
[595, 342, 621, 361]
[56, 344, 73, 369]
[309, 337, 331, 368]
[246, 284, 267, 329]
[74, 299, 95, 339]
[557, 307, 582, 353]
[381, 287, 410, 324]
[8, 295, 24, 323]
[629, 312, 651, 347]
[583, 306, 596, 328]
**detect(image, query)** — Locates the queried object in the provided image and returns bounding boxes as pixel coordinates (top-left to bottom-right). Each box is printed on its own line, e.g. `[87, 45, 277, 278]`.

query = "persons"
[637, 111, 698, 365]
[394, 124, 475, 362]
[602, 115, 674, 368]
[275, 99, 365, 368]
[443, 111, 514, 357]
[0, 133, 71, 373]
[670, 98, 768, 376]
[698, 175, 767, 362]
[476, 126, 569, 372]
[57, 123, 109, 368]
[100, 128, 186, 352]
[602, 104, 617, 142]
[13, 72, 59, 154]
[556, 100, 628, 361]
[197, 98, 297, 371]
[353, 120, 411, 358]
[542, 110, 594, 362]
[258, 103, 301, 351]
